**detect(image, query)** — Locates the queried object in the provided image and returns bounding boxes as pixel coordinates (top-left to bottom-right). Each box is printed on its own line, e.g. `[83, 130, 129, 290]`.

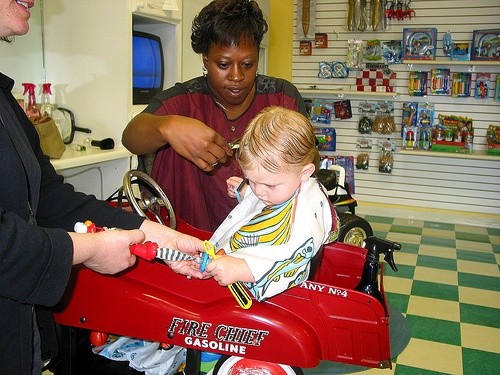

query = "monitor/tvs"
[131, 31, 165, 105]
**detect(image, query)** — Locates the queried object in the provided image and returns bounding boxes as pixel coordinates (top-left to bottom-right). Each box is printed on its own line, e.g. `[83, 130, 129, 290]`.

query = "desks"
[50, 142, 135, 170]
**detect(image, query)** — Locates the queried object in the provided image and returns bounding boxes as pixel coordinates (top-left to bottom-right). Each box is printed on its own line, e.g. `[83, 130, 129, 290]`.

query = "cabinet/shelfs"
[399, 60, 500, 161]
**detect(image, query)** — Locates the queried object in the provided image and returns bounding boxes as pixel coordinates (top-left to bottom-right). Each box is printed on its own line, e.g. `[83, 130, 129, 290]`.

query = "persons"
[0, 0, 215, 375]
[205, 105, 341, 303]
[121, 0, 320, 232]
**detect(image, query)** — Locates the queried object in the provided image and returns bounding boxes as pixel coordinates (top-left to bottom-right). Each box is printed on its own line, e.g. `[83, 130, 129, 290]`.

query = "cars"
[51, 167, 402, 375]
